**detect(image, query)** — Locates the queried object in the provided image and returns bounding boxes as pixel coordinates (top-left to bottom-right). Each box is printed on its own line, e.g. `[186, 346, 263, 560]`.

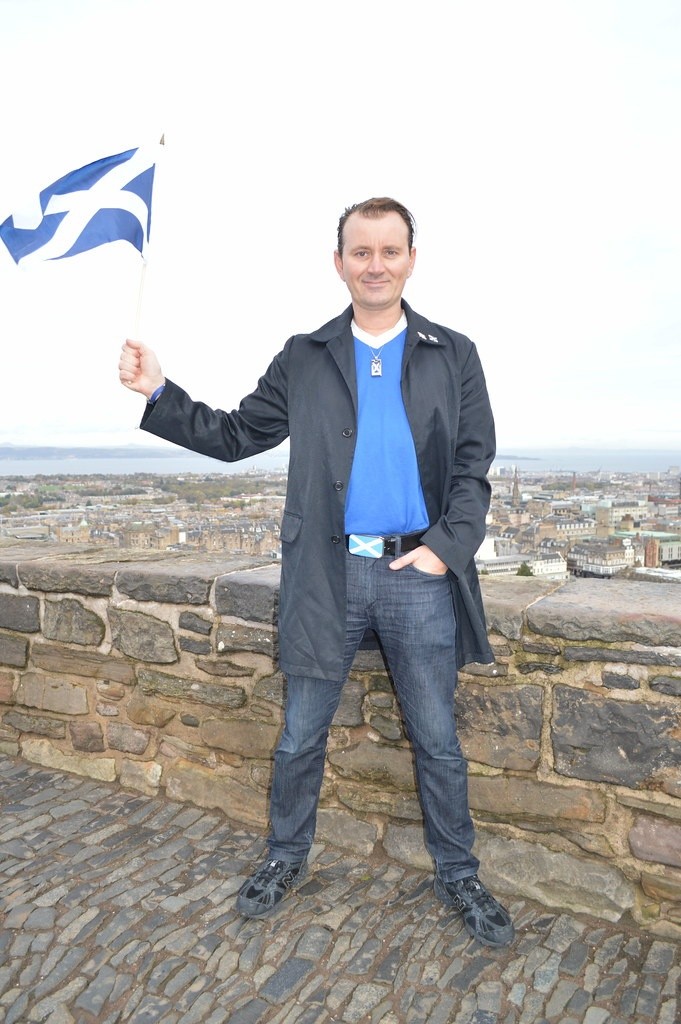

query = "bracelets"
[146, 383, 166, 405]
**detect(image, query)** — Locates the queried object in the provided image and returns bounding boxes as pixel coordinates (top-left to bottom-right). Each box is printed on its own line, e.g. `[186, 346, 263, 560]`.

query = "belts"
[345, 530, 430, 554]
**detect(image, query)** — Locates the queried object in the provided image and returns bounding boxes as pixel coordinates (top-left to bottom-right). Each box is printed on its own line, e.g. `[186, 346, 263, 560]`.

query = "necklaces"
[354, 310, 404, 377]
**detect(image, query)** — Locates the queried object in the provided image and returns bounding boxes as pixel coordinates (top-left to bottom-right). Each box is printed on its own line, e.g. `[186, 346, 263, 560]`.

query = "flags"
[0, 145, 156, 265]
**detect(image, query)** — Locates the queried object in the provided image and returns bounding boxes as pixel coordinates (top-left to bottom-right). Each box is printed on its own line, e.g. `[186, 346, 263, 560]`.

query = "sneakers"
[234, 853, 310, 920]
[433, 874, 516, 946]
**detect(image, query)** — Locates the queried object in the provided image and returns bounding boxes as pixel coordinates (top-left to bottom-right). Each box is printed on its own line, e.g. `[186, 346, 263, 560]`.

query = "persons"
[118, 196, 517, 947]
